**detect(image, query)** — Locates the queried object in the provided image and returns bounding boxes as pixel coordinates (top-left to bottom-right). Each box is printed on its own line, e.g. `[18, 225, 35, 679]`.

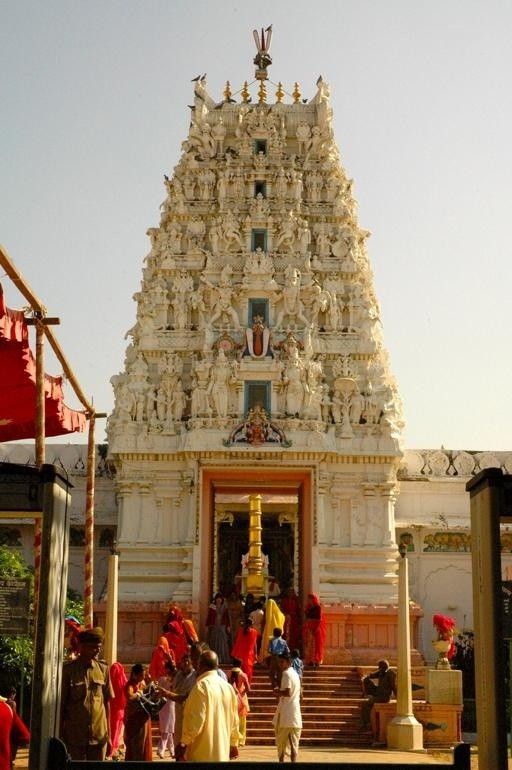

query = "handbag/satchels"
[138, 681, 168, 718]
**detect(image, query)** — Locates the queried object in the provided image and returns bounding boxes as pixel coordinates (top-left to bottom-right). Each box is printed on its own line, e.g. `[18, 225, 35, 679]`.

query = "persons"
[101, 70, 404, 441]
[60, 626, 116, 761]
[267, 627, 304, 762]
[1, 669, 30, 770]
[357, 658, 397, 730]
[205, 577, 325, 684]
[106, 606, 250, 762]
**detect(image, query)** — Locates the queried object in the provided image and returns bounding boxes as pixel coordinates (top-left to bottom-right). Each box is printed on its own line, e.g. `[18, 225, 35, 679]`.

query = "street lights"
[103, 537, 120, 665]
[386, 541, 426, 751]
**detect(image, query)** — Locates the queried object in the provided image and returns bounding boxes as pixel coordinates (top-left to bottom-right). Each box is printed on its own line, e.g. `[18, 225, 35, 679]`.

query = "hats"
[80, 627, 106, 642]
